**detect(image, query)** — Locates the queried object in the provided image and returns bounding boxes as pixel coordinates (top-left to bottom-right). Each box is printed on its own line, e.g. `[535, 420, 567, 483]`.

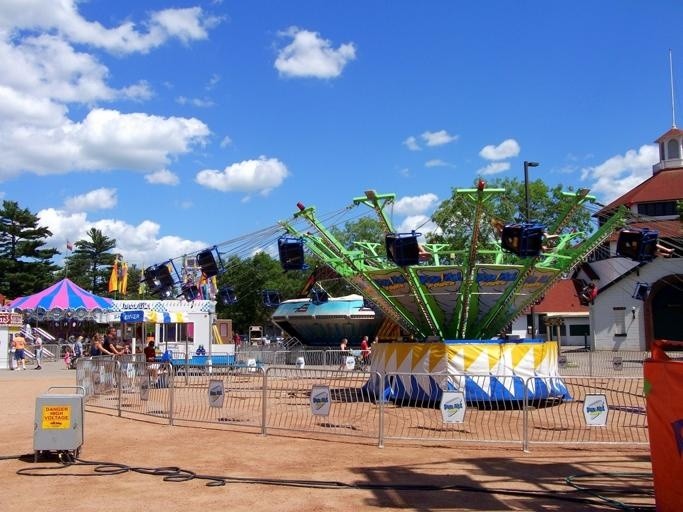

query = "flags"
[108, 258, 145, 295]
[67, 241, 74, 250]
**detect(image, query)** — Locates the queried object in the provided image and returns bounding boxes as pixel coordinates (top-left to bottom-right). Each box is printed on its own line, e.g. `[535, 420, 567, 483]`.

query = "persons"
[182, 278, 201, 300]
[338, 337, 348, 371]
[385, 232, 432, 262]
[505, 223, 560, 252]
[61, 326, 157, 389]
[360, 336, 372, 364]
[232, 331, 242, 353]
[582, 282, 597, 302]
[497, 331, 509, 340]
[619, 228, 676, 257]
[8, 313, 43, 370]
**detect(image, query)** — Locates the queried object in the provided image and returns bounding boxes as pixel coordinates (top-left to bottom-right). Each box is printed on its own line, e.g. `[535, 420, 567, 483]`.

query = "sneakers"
[16, 366, 42, 371]
